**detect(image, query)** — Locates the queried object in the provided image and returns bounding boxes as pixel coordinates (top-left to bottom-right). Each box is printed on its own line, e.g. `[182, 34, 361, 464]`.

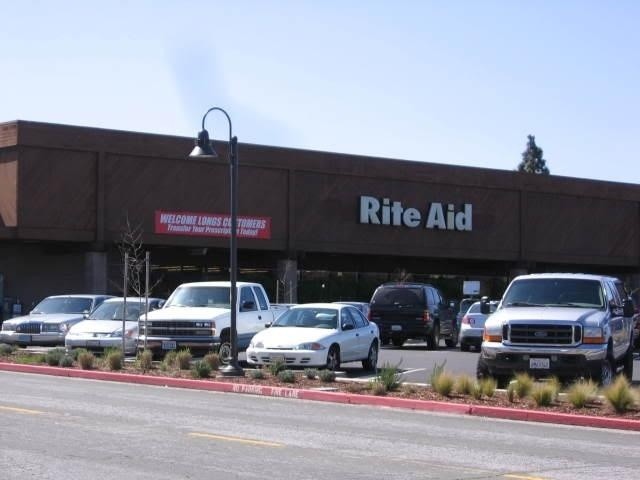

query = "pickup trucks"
[138, 282, 297, 366]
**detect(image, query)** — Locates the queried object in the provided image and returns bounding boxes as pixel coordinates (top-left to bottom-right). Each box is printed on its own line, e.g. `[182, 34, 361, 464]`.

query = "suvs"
[477, 273, 635, 388]
[371, 284, 458, 349]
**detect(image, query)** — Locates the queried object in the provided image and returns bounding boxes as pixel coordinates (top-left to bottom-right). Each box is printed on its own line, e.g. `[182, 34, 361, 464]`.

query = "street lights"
[189, 108, 245, 375]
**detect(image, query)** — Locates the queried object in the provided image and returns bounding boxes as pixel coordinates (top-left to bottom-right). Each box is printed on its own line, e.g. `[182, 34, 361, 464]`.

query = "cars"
[3, 296, 167, 359]
[242, 301, 380, 374]
[459, 298, 500, 351]
